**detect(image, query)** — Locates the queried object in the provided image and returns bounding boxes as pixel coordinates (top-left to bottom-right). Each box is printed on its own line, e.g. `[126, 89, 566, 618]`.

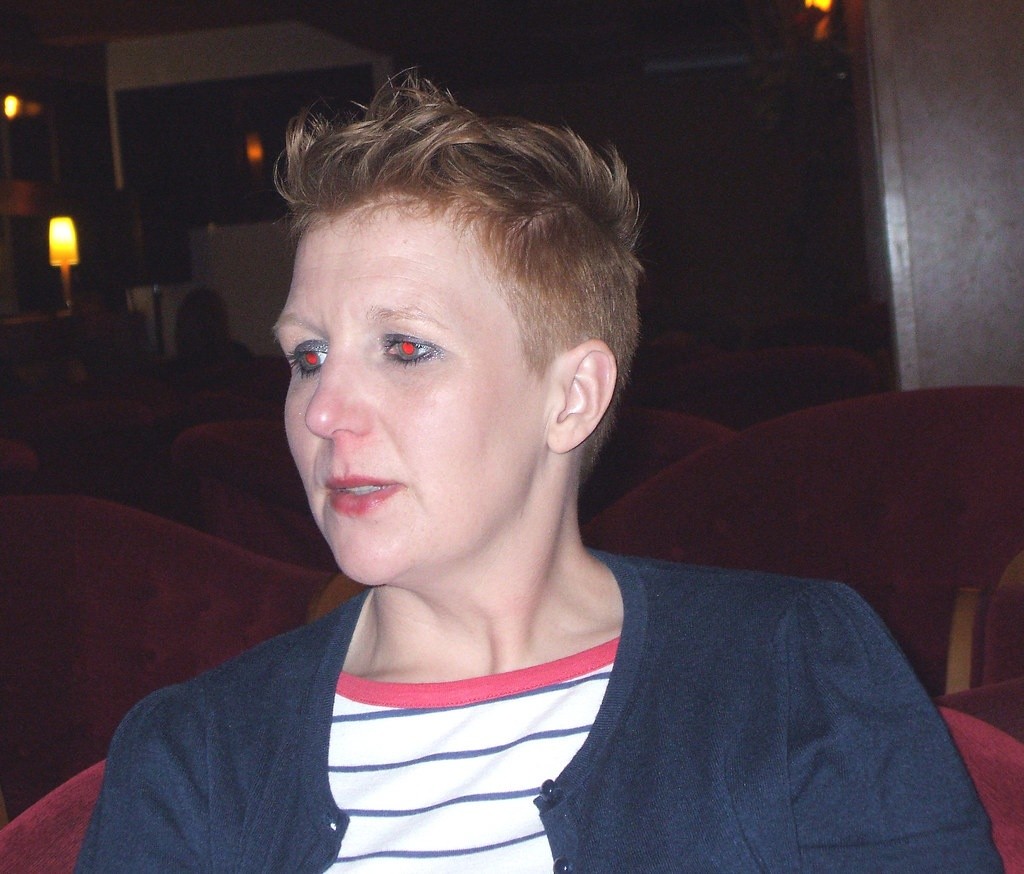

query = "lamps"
[47, 215, 82, 309]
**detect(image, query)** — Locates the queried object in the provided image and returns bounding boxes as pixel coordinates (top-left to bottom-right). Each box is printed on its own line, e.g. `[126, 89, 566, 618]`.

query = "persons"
[72, 70, 1011, 873]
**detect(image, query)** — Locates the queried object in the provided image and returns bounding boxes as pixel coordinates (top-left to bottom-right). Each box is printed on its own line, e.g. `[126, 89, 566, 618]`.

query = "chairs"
[0, 707, 1024, 874]
[581, 330, 1024, 745]
[1, 355, 369, 829]
